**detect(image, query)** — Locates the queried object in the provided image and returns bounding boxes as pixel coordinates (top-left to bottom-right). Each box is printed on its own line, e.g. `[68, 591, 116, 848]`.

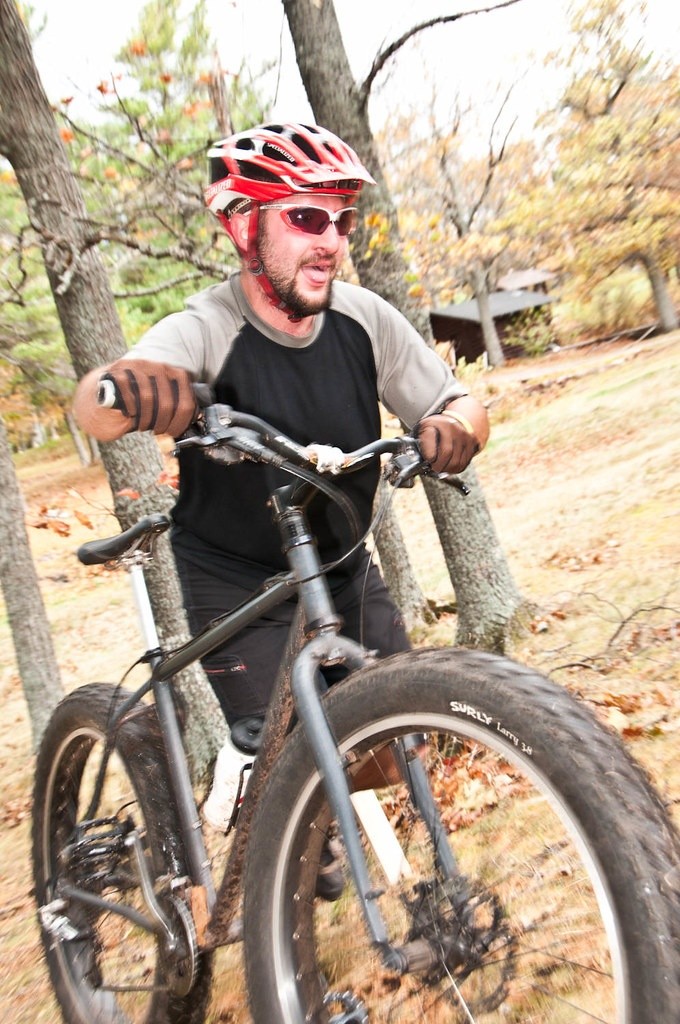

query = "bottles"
[199, 716, 264, 832]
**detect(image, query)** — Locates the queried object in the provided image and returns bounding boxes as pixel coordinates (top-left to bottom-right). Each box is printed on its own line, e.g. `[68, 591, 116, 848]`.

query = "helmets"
[203, 120, 376, 218]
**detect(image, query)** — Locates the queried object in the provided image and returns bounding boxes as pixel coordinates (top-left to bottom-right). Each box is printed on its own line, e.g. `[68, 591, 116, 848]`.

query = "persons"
[65, 119, 492, 901]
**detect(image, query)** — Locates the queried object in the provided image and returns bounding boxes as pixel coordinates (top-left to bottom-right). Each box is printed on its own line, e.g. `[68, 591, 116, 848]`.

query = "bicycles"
[26, 366, 680, 1023]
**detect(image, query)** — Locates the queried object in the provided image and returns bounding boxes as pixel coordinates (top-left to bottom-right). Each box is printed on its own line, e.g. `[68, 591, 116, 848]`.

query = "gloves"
[102, 359, 199, 439]
[411, 411, 480, 475]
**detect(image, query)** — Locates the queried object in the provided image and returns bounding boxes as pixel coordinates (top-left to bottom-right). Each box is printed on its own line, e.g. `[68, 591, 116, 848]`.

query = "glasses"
[259, 202, 358, 237]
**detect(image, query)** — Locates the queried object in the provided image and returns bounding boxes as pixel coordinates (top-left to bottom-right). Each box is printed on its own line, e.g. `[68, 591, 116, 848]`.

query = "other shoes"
[315, 844, 344, 904]
